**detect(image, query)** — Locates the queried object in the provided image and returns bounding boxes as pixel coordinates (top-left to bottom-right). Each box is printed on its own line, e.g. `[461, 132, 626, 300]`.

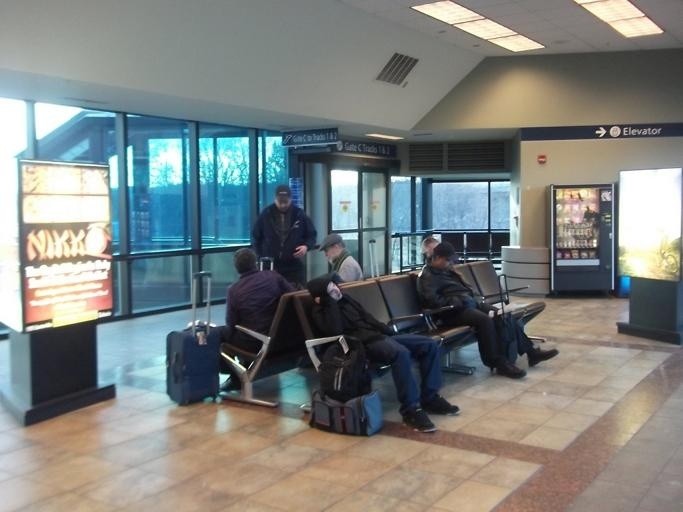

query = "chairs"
[217, 228, 545, 407]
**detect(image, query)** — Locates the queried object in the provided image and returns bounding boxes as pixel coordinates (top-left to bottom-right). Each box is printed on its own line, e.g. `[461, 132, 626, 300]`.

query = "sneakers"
[402, 405, 438, 433]
[220, 376, 242, 392]
[496, 360, 528, 379]
[420, 393, 463, 415]
[528, 348, 559, 367]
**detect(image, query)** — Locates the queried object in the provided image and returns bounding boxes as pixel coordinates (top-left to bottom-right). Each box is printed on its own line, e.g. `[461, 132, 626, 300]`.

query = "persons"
[210, 247, 297, 391]
[415, 241, 559, 378]
[249, 185, 317, 286]
[305, 277, 461, 433]
[421, 237, 439, 259]
[318, 234, 362, 283]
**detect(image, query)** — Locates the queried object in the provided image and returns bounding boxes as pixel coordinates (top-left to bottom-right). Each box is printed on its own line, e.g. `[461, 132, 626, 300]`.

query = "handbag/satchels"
[308, 382, 386, 437]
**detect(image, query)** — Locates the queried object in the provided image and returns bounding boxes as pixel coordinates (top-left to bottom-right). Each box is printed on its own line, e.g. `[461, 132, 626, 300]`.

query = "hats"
[318, 234, 342, 252]
[276, 185, 292, 196]
[433, 241, 460, 261]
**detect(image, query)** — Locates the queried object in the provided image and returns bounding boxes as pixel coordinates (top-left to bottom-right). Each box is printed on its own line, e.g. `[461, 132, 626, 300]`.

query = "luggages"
[165, 319, 220, 406]
[476, 307, 516, 367]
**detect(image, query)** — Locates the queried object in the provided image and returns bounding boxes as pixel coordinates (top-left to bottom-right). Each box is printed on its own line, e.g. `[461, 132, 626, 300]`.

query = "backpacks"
[317, 333, 372, 403]
